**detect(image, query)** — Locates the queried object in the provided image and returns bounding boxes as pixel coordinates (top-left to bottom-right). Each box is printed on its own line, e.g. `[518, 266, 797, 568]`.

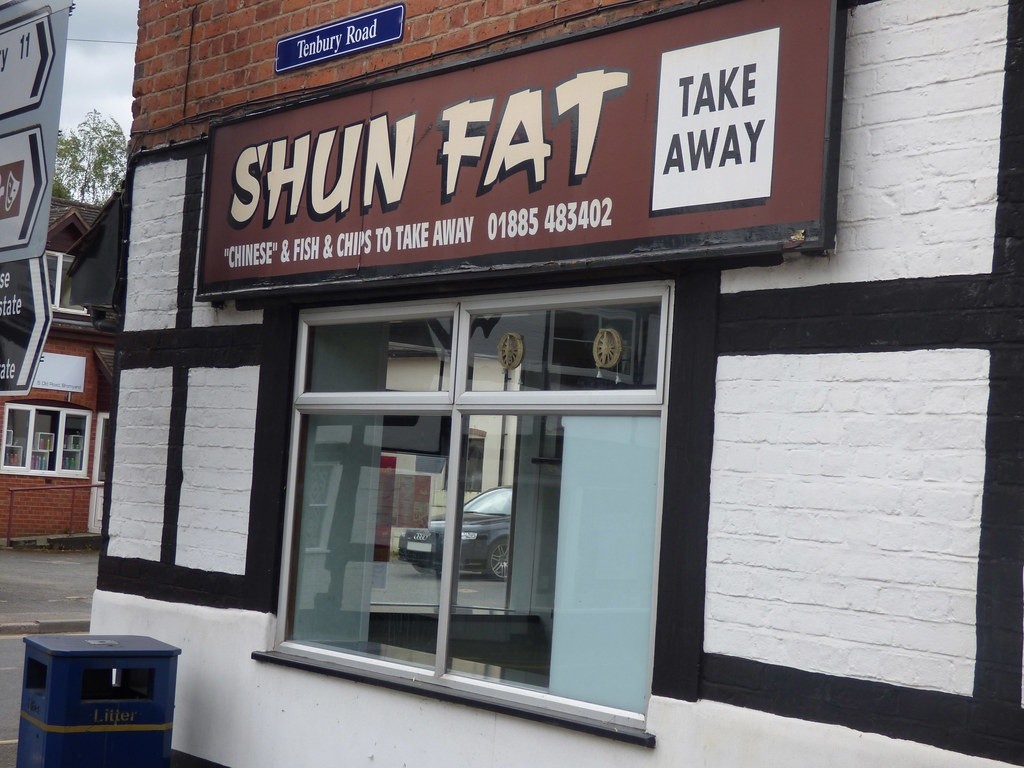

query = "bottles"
[46, 437, 50, 450]
[76, 442, 80, 448]
[6, 451, 18, 465]
[31, 454, 46, 470]
[64, 456, 70, 469]
[70, 456, 75, 468]
[40, 437, 45, 449]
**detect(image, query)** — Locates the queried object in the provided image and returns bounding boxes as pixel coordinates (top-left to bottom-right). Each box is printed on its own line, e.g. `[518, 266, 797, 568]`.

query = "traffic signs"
[0, 0, 77, 395]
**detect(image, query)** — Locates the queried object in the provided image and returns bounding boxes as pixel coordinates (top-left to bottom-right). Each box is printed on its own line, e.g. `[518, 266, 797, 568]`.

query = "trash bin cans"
[16, 634, 182, 768]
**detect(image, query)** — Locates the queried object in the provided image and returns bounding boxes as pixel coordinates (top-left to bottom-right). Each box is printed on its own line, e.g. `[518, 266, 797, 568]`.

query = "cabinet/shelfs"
[4, 430, 23, 466]
[63, 434, 84, 470]
[31, 432, 55, 471]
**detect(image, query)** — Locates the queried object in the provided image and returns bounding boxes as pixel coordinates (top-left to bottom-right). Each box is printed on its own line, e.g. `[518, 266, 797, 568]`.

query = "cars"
[398, 484, 513, 582]
